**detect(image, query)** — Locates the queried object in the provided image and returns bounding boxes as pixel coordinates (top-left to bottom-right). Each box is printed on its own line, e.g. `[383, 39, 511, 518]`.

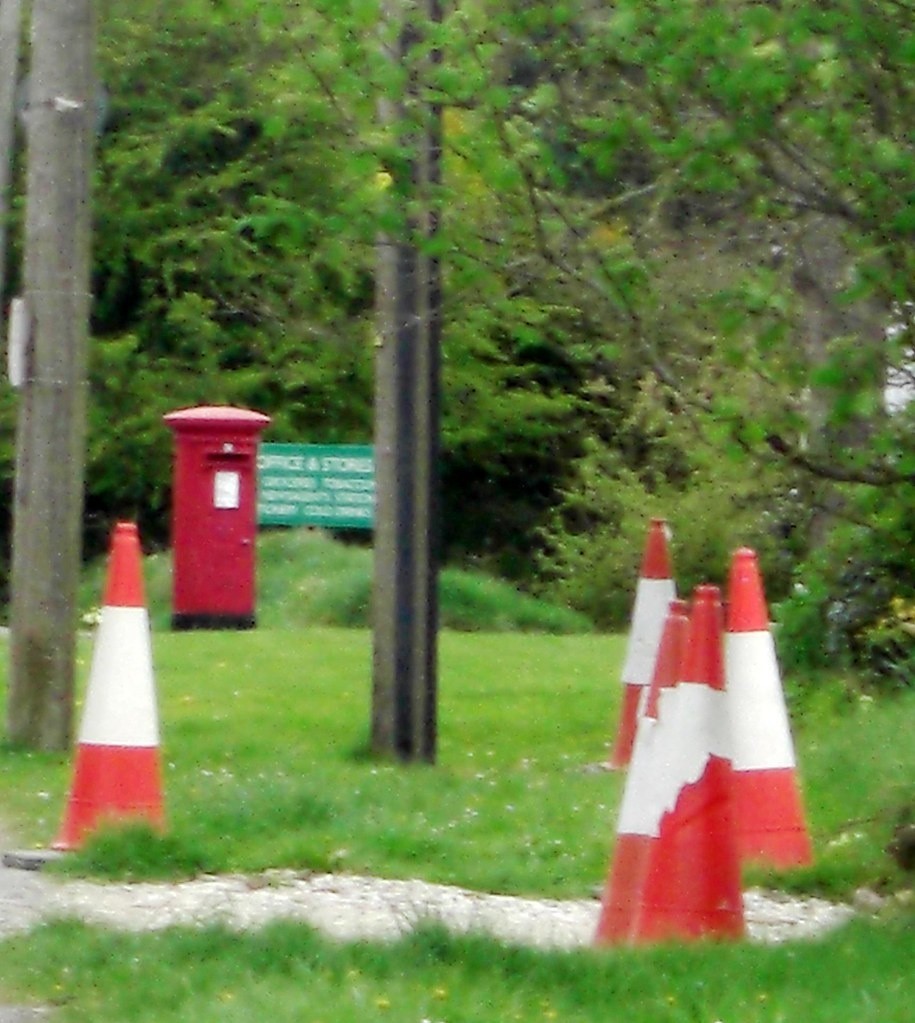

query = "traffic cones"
[597, 597, 689, 946]
[633, 583, 745, 944]
[55, 515, 163, 852]
[724, 545, 811, 866]
[602, 519, 679, 770]
